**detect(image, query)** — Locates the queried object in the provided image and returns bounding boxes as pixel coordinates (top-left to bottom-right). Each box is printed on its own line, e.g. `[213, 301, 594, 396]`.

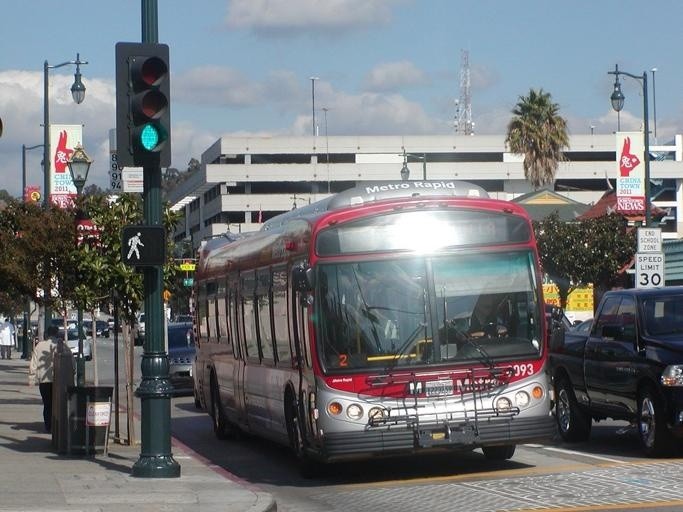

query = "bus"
[191, 180, 555, 479]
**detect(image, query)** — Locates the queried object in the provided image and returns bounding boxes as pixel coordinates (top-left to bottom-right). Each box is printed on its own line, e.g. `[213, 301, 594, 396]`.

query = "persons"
[0, 318, 16, 360]
[453, 294, 511, 350]
[27, 324, 77, 435]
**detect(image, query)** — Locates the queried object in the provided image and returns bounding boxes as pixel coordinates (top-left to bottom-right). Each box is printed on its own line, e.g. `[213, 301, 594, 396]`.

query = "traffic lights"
[115, 41, 171, 168]
[122, 225, 167, 267]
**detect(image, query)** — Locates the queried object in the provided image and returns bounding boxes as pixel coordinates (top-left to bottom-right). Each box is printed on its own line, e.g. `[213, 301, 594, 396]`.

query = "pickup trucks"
[546, 285, 683, 457]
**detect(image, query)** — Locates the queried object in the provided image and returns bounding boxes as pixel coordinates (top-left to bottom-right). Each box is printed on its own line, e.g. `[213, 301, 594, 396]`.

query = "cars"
[113, 313, 145, 346]
[546, 304, 572, 342]
[167, 314, 199, 397]
[12, 318, 114, 361]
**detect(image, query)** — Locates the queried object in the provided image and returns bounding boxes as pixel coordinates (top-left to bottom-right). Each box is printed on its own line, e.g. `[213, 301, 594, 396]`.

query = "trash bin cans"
[65, 386, 113, 455]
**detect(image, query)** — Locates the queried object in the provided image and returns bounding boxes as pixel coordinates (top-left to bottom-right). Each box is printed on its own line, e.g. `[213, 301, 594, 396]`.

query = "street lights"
[398, 149, 426, 181]
[22, 145, 44, 199]
[607, 62, 651, 228]
[44, 53, 87, 342]
[67, 143, 94, 387]
